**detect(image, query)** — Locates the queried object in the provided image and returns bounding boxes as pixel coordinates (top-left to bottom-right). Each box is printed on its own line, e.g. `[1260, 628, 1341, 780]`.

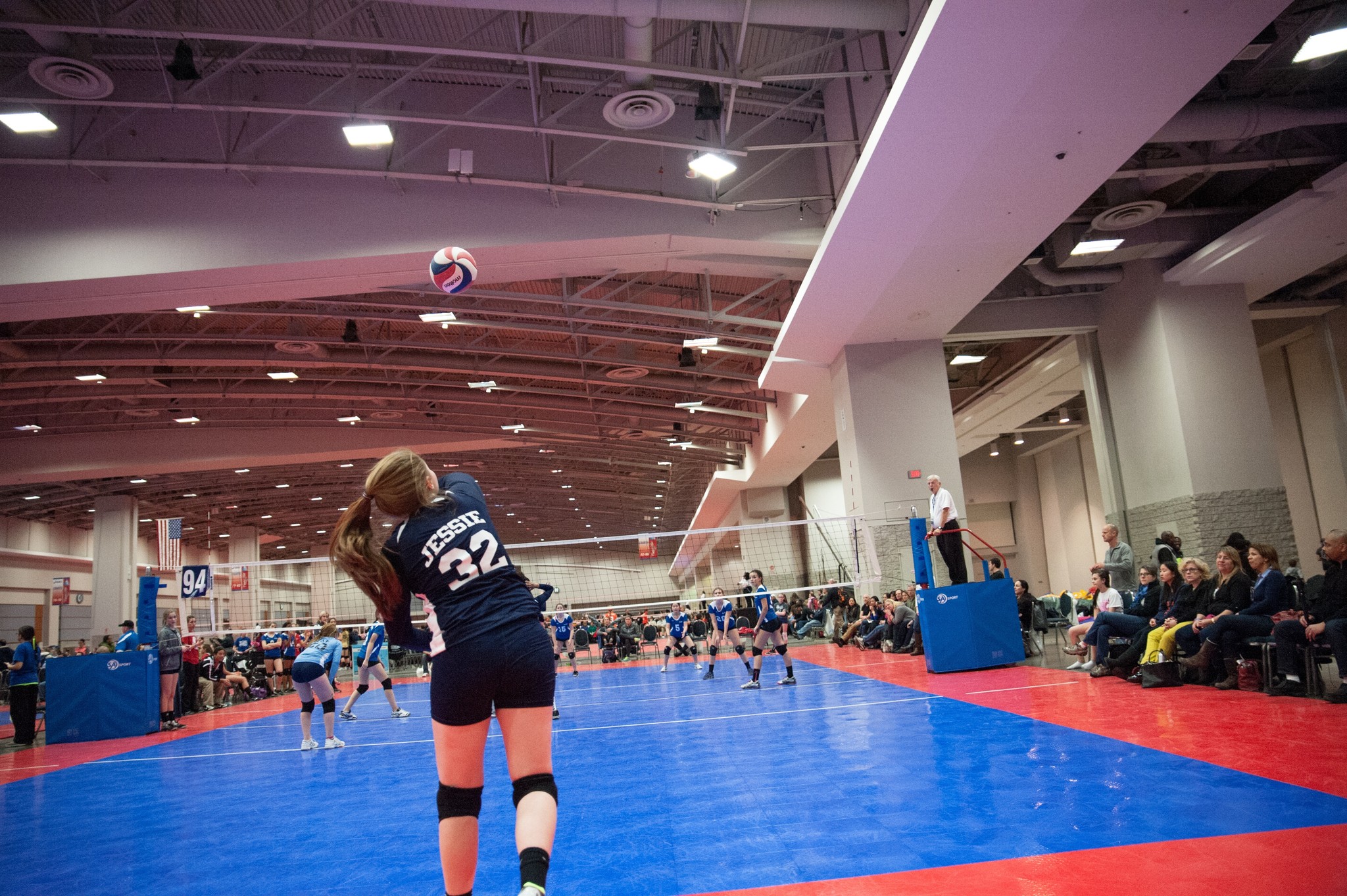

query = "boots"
[1103, 646, 1138, 680]
[910, 634, 923, 656]
[1177, 637, 1238, 689]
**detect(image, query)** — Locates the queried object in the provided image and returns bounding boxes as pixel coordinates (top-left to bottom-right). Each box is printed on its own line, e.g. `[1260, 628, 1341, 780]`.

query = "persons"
[517, 569, 956, 719]
[989, 557, 1004, 580]
[1014, 579, 1032, 638]
[0, 607, 433, 751]
[1062, 524, 1347, 704]
[926, 475, 967, 585]
[327, 447, 558, 896]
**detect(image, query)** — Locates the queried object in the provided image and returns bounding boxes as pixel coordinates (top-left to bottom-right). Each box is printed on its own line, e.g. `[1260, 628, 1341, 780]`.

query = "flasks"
[1159, 648, 1164, 663]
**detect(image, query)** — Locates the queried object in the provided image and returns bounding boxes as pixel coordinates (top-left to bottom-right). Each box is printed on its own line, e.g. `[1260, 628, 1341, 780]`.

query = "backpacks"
[601, 648, 618, 663]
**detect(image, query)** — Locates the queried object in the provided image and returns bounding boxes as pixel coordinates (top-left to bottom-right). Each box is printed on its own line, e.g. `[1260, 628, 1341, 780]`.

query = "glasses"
[1249, 586, 1255, 602]
[1138, 573, 1151, 577]
[1182, 568, 1199, 573]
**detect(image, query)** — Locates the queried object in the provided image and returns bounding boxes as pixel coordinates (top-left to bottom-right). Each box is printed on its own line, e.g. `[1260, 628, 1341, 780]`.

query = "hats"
[118, 620, 134, 628]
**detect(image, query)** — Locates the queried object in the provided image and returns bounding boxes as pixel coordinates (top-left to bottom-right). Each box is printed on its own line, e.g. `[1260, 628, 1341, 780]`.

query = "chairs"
[34, 669, 46, 739]
[597, 625, 660, 661]
[559, 629, 592, 665]
[673, 616, 753, 655]
[810, 611, 828, 641]
[1022, 580, 1331, 696]
[223, 655, 271, 704]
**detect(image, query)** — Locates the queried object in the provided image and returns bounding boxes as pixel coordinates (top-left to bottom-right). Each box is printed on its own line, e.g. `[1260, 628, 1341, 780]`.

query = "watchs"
[937, 526, 943, 530]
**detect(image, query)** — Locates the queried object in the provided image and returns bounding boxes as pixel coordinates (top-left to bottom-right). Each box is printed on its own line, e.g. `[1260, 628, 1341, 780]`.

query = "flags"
[156, 517, 181, 571]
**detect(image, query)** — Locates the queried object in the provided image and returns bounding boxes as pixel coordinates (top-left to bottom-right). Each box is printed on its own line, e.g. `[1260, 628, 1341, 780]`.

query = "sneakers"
[740, 676, 796, 689]
[1062, 642, 1111, 677]
[703, 667, 753, 680]
[203, 702, 227, 711]
[390, 707, 411, 718]
[301, 735, 345, 750]
[301, 746, 344, 760]
[338, 709, 357, 720]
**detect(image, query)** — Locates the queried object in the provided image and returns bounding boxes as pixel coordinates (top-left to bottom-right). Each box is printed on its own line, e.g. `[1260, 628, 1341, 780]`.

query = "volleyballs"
[429, 246, 478, 295]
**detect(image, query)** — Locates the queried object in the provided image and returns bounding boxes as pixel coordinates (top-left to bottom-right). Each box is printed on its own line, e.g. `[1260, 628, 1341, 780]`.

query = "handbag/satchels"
[1140, 649, 1183, 688]
[244, 684, 268, 701]
[1236, 656, 1265, 692]
[1270, 607, 1307, 637]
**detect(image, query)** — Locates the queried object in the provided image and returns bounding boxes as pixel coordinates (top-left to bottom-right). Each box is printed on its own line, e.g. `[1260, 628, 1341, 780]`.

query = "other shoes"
[1265, 678, 1347, 704]
[832, 635, 914, 651]
[249, 688, 297, 702]
[160, 719, 186, 731]
[661, 663, 703, 672]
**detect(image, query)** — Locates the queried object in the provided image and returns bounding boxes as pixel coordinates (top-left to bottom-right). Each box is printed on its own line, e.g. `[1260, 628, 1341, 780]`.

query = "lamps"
[693, 85, 721, 122]
[679, 348, 696, 367]
[166, 42, 202, 82]
[342, 319, 360, 343]
